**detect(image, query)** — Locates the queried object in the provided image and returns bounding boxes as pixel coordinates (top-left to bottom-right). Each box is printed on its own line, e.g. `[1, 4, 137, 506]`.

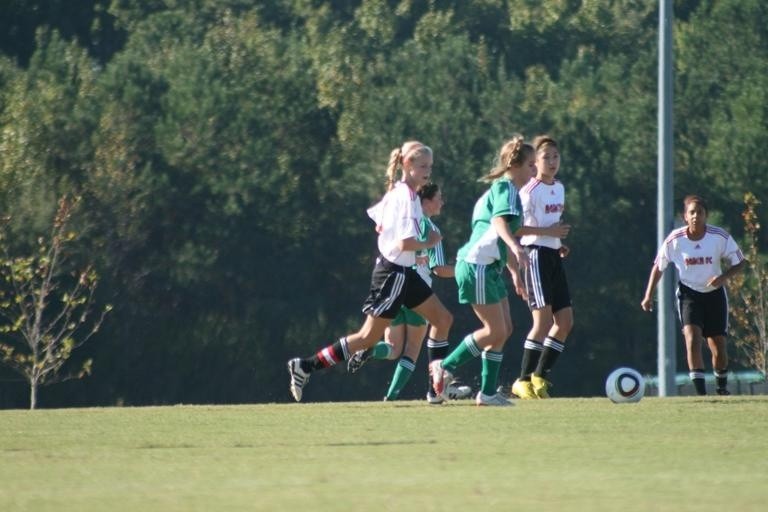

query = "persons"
[287, 140, 473, 404]
[510, 135, 574, 400]
[345, 181, 457, 397]
[427, 135, 534, 407]
[641, 194, 745, 396]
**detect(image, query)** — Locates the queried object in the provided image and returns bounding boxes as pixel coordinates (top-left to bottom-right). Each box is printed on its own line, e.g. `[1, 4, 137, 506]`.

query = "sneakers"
[477, 390, 514, 406]
[511, 378, 537, 400]
[427, 384, 471, 404]
[428, 360, 452, 404]
[288, 357, 311, 401]
[530, 372, 550, 398]
[347, 347, 373, 375]
[716, 388, 730, 395]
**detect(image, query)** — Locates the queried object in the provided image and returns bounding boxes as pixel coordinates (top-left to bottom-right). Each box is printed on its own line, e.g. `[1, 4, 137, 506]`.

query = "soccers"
[605, 367, 645, 404]
